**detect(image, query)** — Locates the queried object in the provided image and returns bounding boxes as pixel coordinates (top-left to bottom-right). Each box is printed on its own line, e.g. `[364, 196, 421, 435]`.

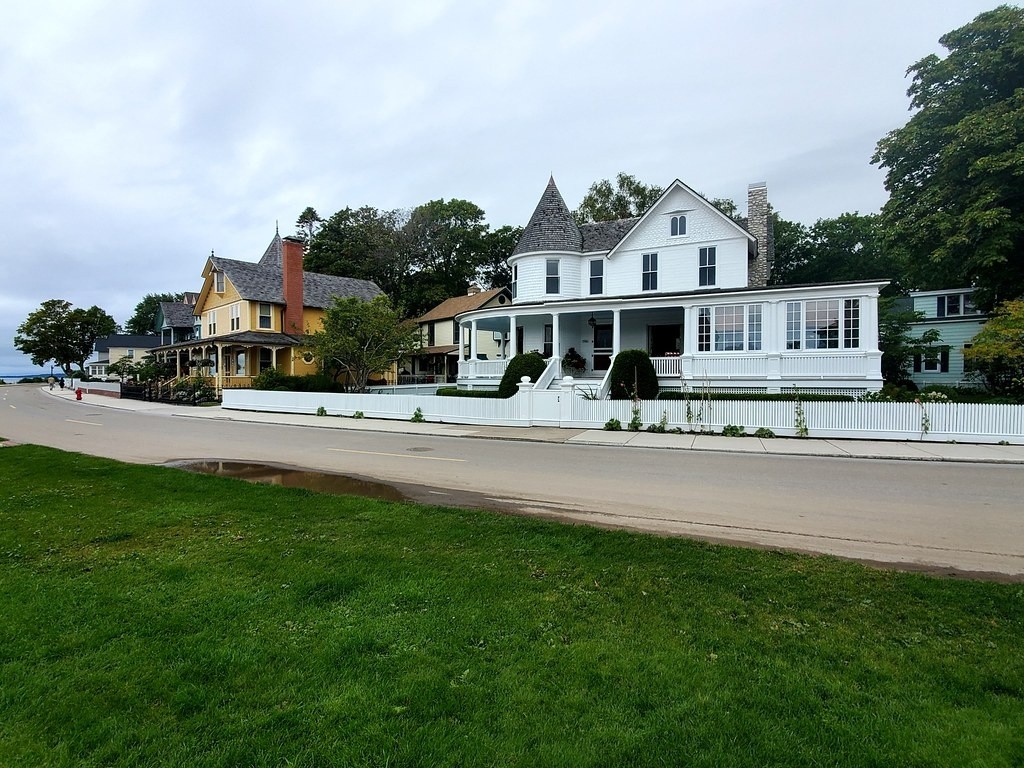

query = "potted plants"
[560, 346, 587, 379]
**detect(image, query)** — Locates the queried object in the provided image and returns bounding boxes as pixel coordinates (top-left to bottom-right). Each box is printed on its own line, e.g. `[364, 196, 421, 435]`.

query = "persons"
[48, 375, 55, 390]
[60, 377, 65, 391]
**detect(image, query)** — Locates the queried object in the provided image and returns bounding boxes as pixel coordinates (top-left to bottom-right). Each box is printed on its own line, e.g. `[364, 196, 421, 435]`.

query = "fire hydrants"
[74, 387, 82, 400]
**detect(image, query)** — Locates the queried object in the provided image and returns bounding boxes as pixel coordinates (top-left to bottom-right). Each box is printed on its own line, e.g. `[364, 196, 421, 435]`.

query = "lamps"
[588, 312, 596, 328]
